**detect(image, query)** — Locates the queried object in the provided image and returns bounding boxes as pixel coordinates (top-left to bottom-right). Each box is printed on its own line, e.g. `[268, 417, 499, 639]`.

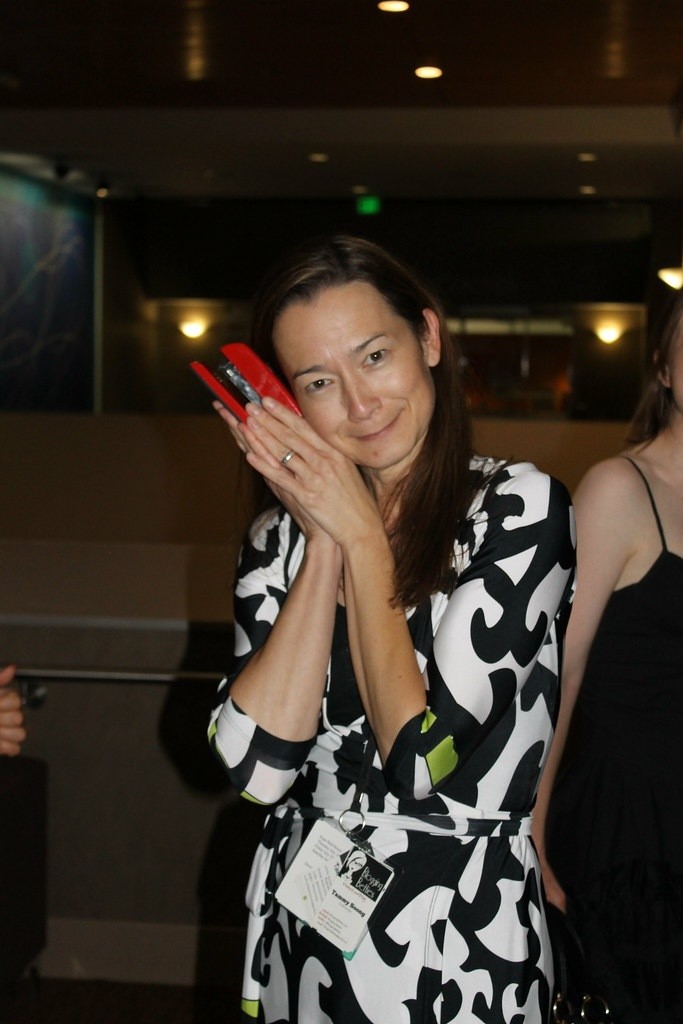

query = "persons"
[201, 238, 570, 1024]
[519, 295, 681, 1023]
[2, 667, 51, 1019]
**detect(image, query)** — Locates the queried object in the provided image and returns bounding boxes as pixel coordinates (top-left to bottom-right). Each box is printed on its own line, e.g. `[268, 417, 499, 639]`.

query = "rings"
[281, 449, 295, 464]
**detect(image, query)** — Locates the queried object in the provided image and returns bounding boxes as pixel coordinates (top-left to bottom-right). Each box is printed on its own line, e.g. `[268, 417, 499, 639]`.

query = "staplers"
[192, 342, 303, 430]
